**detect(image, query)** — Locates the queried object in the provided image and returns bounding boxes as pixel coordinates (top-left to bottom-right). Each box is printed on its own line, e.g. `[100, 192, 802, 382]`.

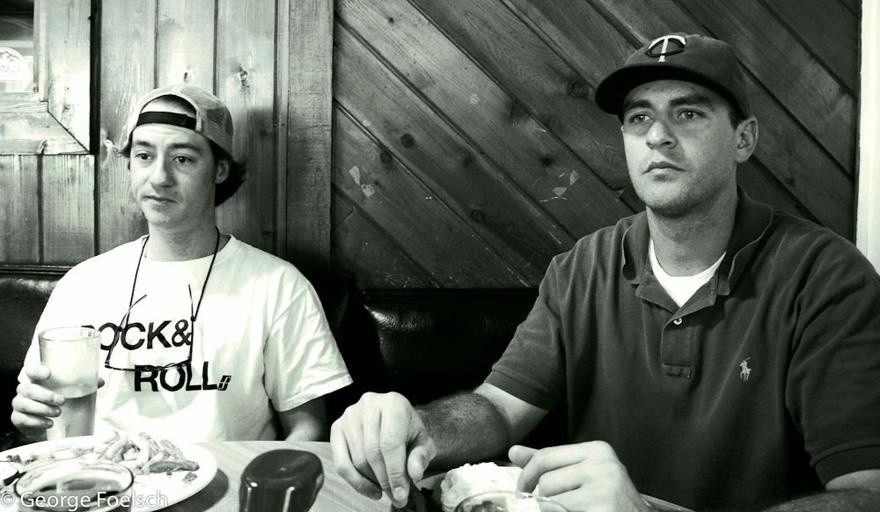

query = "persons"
[327, 22, 880, 511]
[6, 80, 357, 446]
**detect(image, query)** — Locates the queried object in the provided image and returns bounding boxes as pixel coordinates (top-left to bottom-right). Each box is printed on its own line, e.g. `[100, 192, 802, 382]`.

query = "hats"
[118, 80, 234, 156]
[595, 33, 750, 121]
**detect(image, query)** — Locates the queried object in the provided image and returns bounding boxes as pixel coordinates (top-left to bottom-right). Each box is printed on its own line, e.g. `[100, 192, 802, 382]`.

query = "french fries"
[90, 427, 200, 475]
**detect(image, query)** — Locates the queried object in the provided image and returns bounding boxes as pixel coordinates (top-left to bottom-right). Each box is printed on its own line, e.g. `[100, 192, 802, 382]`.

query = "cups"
[37, 326, 103, 439]
[15, 457, 133, 512]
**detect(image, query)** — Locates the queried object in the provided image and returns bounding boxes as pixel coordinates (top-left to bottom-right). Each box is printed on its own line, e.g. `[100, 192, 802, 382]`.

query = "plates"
[0, 433, 218, 512]
[388, 465, 699, 512]
